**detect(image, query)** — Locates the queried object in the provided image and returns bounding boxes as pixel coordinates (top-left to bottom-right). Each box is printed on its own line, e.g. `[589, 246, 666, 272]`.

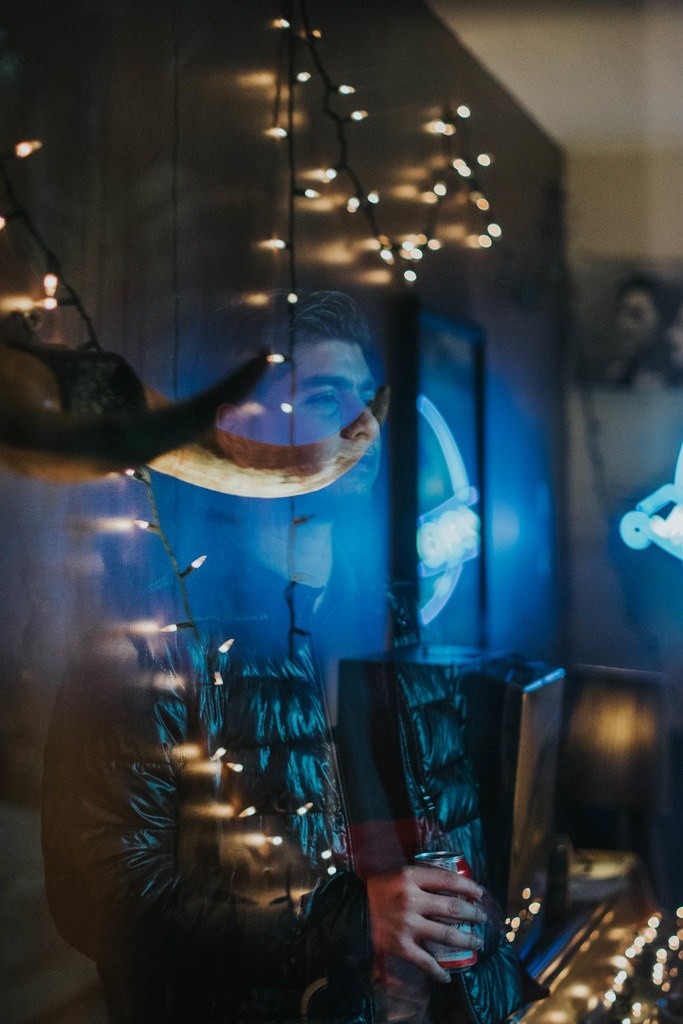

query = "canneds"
[413, 850, 479, 974]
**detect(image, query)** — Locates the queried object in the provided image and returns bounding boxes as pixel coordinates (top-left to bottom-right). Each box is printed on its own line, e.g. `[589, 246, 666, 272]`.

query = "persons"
[568, 279, 683, 662]
[43, 294, 552, 1022]
